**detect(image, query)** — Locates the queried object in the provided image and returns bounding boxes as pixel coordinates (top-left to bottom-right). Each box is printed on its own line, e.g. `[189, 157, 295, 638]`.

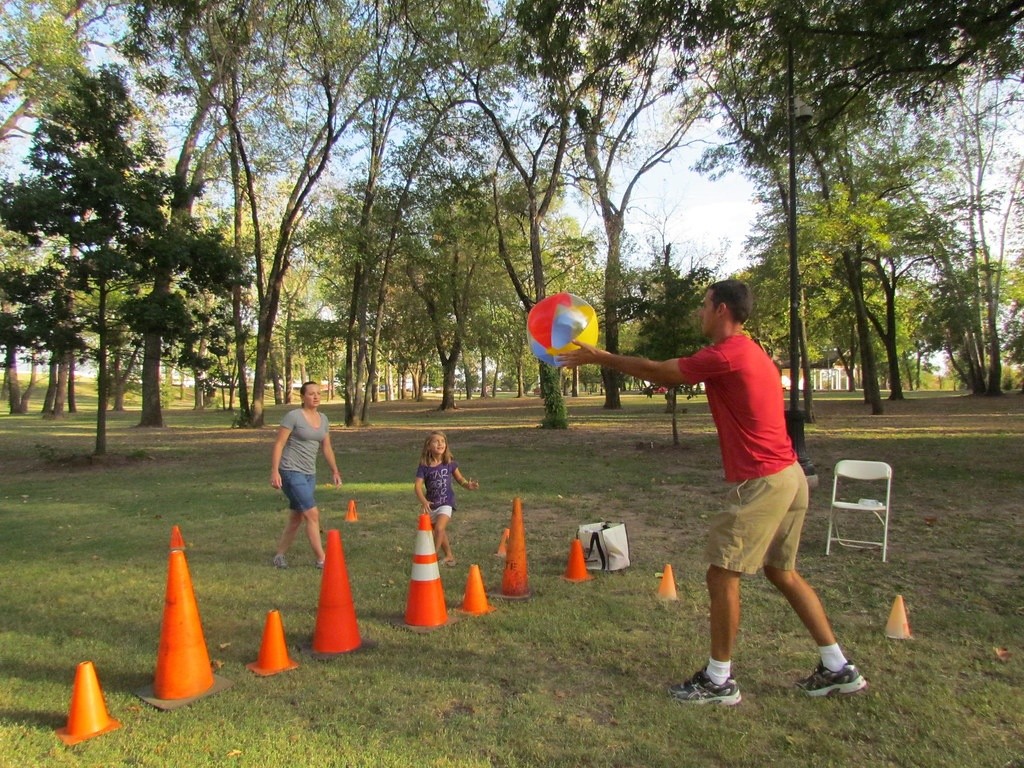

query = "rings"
[272, 483, 273, 485]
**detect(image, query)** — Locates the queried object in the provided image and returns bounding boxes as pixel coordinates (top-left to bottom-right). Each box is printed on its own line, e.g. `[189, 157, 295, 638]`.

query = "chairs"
[825, 460, 892, 563]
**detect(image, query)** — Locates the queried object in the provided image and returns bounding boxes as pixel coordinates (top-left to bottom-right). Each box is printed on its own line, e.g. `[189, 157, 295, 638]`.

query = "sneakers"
[315, 559, 324, 569]
[795, 656, 867, 697]
[666, 666, 742, 706]
[273, 554, 289, 569]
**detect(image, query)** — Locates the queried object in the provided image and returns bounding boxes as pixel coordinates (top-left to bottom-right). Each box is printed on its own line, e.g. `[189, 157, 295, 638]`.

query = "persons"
[271, 381, 343, 569]
[557, 280, 866, 705]
[414, 430, 480, 567]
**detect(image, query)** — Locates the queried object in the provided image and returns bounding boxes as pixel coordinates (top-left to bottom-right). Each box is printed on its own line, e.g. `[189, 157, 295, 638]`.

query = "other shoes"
[437, 556, 458, 566]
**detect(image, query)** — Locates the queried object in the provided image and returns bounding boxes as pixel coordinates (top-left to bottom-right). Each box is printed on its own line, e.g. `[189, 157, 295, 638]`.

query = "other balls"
[527, 293, 598, 367]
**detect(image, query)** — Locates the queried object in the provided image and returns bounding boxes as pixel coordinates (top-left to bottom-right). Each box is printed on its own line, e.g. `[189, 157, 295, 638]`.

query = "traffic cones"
[455, 562, 497, 615]
[125, 549, 233, 710]
[563, 537, 594, 582]
[392, 513, 459, 634]
[492, 495, 533, 600]
[246, 610, 299, 678]
[296, 528, 379, 661]
[493, 533, 513, 559]
[656, 562, 678, 602]
[168, 524, 187, 551]
[885, 593, 911, 640]
[54, 662, 123, 747]
[346, 499, 358, 522]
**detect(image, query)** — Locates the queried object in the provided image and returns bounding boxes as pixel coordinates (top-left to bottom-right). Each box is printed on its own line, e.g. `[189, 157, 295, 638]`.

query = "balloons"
[526, 294, 599, 367]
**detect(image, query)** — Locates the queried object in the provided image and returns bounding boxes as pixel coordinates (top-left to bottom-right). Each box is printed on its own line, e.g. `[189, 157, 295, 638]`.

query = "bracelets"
[333, 472, 342, 476]
[461, 480, 467, 486]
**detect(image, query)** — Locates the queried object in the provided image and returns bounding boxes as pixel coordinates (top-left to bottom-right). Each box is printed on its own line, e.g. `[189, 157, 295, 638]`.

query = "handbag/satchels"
[574, 521, 631, 572]
